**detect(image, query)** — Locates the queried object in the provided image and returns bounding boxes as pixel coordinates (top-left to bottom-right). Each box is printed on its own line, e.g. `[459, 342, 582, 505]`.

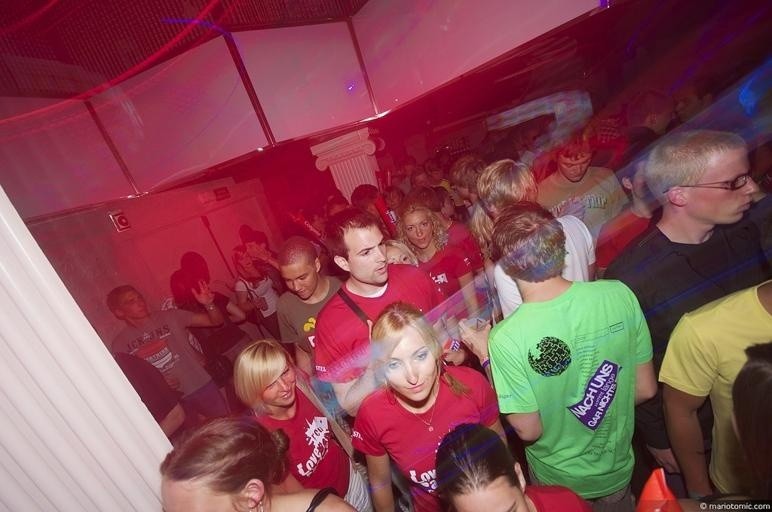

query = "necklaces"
[392, 388, 440, 433]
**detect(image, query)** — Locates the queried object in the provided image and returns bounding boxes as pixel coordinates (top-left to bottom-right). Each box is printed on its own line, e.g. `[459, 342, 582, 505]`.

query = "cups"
[255, 296, 268, 311]
[465, 318, 486, 330]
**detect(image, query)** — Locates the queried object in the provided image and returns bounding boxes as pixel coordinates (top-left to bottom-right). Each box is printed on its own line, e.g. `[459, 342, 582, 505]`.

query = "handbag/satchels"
[244, 307, 266, 326]
[352, 455, 370, 489]
[202, 352, 234, 384]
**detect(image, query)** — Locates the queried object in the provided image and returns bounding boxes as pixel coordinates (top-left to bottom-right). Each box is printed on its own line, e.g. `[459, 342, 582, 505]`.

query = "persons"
[537, 120, 630, 236]
[111, 350, 186, 438]
[351, 305, 506, 512]
[275, 235, 343, 340]
[406, 186, 484, 275]
[233, 244, 289, 341]
[672, 338, 772, 510]
[395, 203, 479, 323]
[382, 235, 423, 270]
[609, 91, 674, 168]
[598, 157, 660, 282]
[241, 231, 281, 261]
[316, 207, 465, 414]
[160, 418, 353, 512]
[351, 105, 544, 232]
[657, 278, 772, 500]
[107, 284, 230, 426]
[483, 200, 656, 512]
[674, 79, 756, 159]
[169, 268, 253, 362]
[478, 158, 597, 319]
[234, 341, 371, 511]
[433, 422, 592, 512]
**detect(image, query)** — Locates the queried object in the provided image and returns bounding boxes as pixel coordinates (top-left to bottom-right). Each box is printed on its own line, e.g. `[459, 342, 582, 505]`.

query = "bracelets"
[203, 304, 216, 313]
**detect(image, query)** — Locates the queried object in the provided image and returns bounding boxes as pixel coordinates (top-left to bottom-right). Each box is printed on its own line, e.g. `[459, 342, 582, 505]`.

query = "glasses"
[662, 169, 755, 194]
